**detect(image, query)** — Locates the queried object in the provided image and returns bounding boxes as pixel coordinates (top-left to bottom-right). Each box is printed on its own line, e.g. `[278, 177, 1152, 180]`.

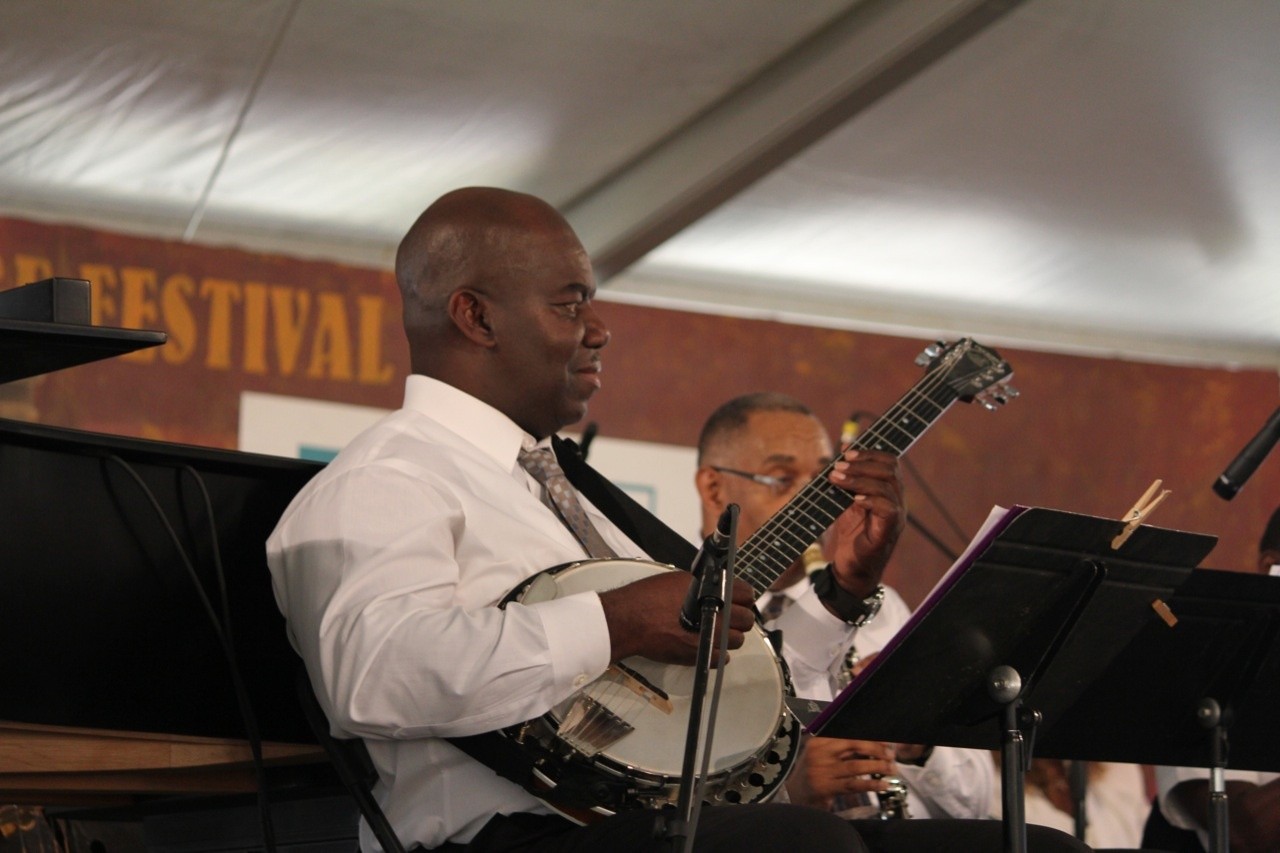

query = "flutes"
[837, 641, 910, 820]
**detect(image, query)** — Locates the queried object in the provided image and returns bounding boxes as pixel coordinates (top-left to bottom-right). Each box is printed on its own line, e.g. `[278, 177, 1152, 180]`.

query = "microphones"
[1213, 408, 1280, 500]
[679, 504, 740, 634]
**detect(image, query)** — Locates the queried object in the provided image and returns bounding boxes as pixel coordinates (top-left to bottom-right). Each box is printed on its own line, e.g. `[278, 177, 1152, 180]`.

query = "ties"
[518, 448, 617, 561]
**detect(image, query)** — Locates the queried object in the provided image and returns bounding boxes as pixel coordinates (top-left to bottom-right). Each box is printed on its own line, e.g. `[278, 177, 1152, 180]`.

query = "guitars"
[488, 332, 1021, 811]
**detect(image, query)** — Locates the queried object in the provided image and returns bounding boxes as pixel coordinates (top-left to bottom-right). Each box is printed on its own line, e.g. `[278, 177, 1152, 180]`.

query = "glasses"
[709, 464, 801, 493]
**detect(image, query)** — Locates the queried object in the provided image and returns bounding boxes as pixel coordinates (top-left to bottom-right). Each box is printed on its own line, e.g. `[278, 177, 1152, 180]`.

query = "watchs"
[815, 562, 885, 624]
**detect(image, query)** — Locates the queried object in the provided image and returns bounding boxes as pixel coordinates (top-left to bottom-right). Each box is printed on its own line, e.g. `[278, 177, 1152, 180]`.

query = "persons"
[697, 391, 993, 817]
[267, 188, 908, 853]
[994, 752, 1279, 853]
